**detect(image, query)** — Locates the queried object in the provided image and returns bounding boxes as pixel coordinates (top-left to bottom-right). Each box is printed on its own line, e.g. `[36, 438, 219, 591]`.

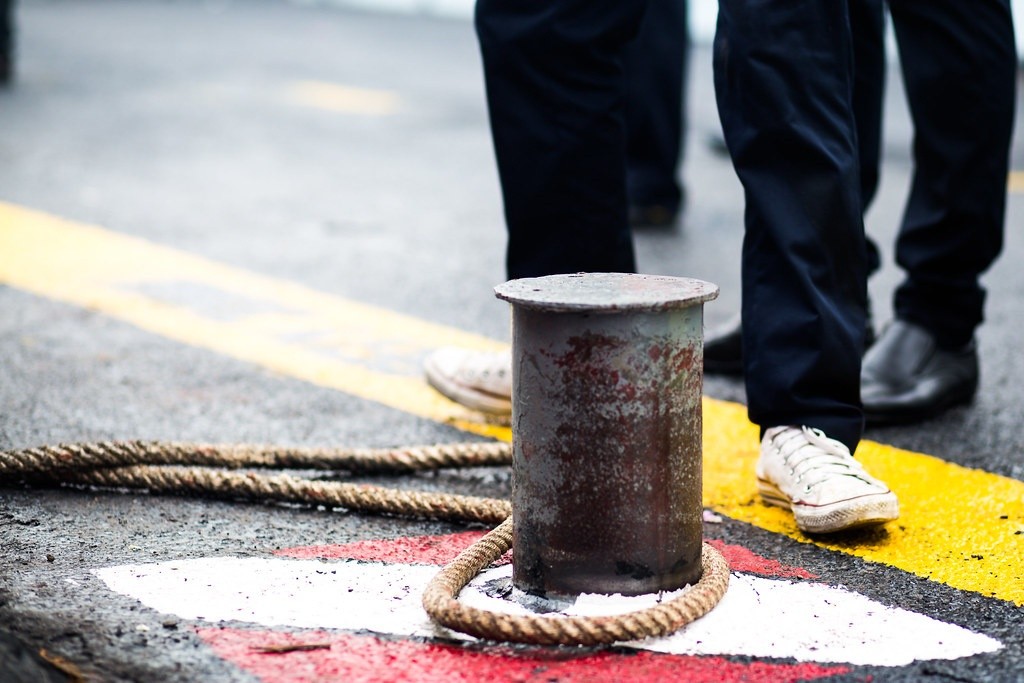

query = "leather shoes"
[856, 316, 981, 425]
[695, 324, 748, 377]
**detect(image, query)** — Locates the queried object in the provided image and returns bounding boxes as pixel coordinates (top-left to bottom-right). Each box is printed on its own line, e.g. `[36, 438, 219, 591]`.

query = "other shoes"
[629, 197, 682, 235]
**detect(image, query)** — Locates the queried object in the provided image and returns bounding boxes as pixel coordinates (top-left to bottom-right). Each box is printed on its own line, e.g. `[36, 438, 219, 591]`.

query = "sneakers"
[421, 349, 515, 408]
[756, 426, 900, 535]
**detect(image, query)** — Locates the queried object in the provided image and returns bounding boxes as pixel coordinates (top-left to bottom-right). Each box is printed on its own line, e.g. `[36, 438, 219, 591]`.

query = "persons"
[619, 0, 688, 229]
[426, 0, 898, 532]
[700, 0, 1018, 426]
